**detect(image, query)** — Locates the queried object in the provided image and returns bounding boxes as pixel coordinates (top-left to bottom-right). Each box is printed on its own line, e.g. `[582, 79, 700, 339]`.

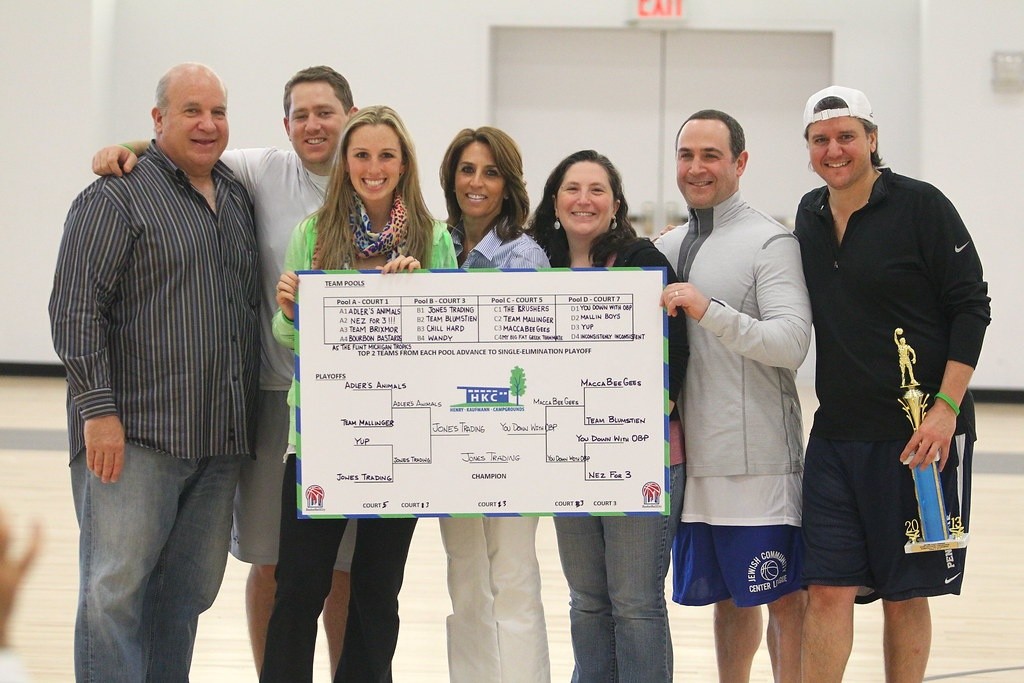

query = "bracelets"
[120, 142, 141, 158]
[935, 393, 961, 417]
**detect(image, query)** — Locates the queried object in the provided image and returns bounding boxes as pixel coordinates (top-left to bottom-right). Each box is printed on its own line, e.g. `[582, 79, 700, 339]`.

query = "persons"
[260, 104, 461, 681]
[656, 109, 815, 683]
[521, 152, 687, 683]
[48, 62, 266, 682]
[437, 127, 553, 683]
[89, 66, 364, 683]
[649, 85, 994, 683]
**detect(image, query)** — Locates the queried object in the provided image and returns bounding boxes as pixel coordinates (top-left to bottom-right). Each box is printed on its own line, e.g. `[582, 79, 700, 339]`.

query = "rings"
[675, 292, 680, 297]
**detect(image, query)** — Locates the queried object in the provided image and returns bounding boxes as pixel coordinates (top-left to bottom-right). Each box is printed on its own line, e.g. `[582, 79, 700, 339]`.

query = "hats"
[805, 85, 876, 132]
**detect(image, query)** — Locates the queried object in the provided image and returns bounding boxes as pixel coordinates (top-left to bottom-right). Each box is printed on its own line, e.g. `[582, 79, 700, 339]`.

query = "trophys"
[891, 325, 972, 553]
